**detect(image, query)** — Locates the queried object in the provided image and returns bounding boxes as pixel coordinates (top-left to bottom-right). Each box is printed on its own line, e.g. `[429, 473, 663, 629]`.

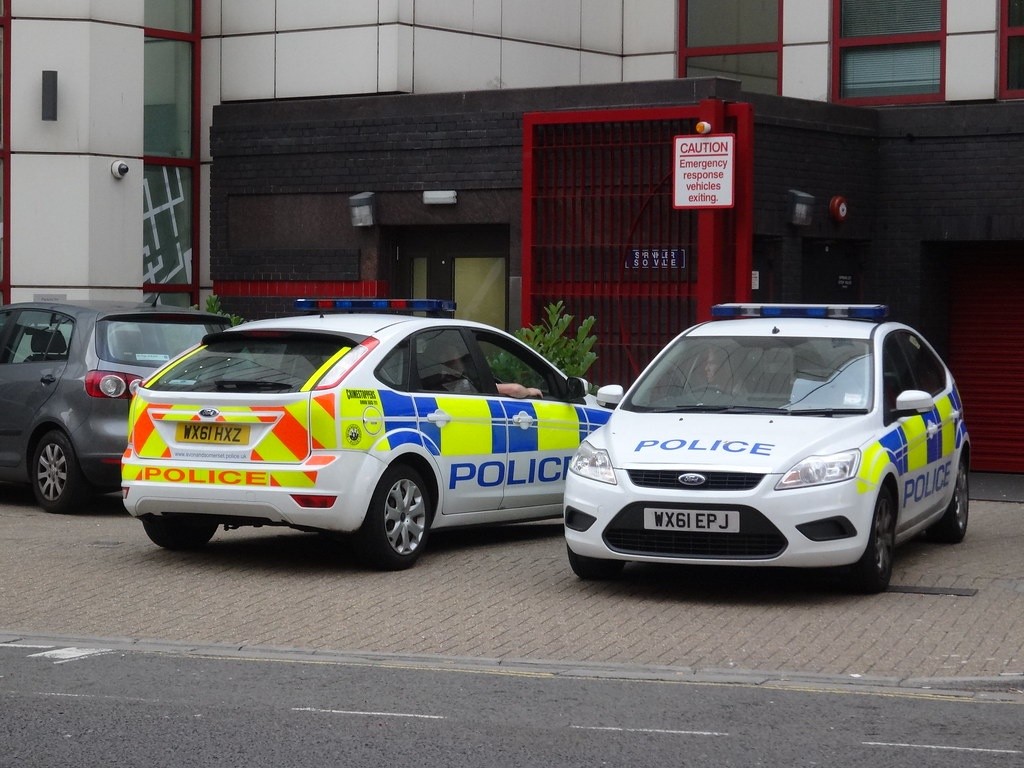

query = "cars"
[560, 303, 972, 596]
[117, 294, 617, 571]
[0, 299, 260, 516]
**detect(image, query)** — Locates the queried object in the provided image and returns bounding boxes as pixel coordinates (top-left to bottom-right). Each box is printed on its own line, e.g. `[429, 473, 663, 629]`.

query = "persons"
[430, 349, 545, 400]
